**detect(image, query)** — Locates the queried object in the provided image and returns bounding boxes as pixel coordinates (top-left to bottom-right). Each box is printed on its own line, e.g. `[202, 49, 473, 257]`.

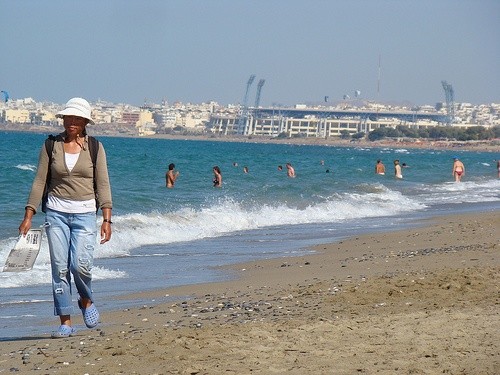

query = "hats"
[55, 98, 96, 125]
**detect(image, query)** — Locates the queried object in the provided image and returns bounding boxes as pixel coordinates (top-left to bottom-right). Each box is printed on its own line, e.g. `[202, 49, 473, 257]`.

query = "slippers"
[77, 296, 100, 328]
[50, 325, 78, 338]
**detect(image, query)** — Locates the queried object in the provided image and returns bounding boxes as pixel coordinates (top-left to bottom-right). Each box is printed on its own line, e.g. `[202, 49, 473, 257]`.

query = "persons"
[452, 157, 464, 182]
[394, 160, 402, 178]
[243, 167, 248, 173]
[286, 162, 295, 177]
[497, 160, 500, 179]
[19, 98, 113, 337]
[166, 163, 179, 188]
[278, 166, 282, 170]
[376, 160, 385, 175]
[213, 166, 222, 187]
[402, 163, 408, 167]
[234, 162, 238, 166]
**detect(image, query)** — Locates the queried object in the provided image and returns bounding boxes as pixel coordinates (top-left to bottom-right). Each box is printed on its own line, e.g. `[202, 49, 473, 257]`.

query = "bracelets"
[104, 219, 112, 224]
[27, 208, 33, 211]
